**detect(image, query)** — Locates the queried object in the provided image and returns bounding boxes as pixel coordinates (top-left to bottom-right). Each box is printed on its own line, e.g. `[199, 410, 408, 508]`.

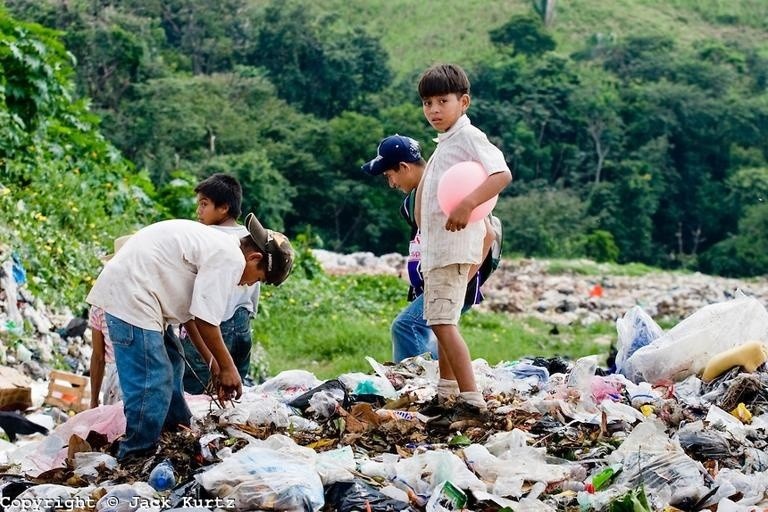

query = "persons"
[181, 174, 261, 398]
[85, 211, 292, 464]
[359, 133, 502, 363]
[414, 64, 513, 434]
[87, 236, 140, 408]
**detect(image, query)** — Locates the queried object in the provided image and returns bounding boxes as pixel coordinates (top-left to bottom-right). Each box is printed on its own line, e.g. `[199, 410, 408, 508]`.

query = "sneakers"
[417, 394, 488, 433]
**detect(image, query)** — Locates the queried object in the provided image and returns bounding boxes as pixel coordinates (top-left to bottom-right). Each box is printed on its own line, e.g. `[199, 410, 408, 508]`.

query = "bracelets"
[209, 356, 213, 374]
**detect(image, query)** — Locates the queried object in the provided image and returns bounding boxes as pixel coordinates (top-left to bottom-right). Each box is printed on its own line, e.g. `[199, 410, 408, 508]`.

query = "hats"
[244, 212, 294, 286]
[362, 133, 422, 175]
[101, 236, 132, 260]
